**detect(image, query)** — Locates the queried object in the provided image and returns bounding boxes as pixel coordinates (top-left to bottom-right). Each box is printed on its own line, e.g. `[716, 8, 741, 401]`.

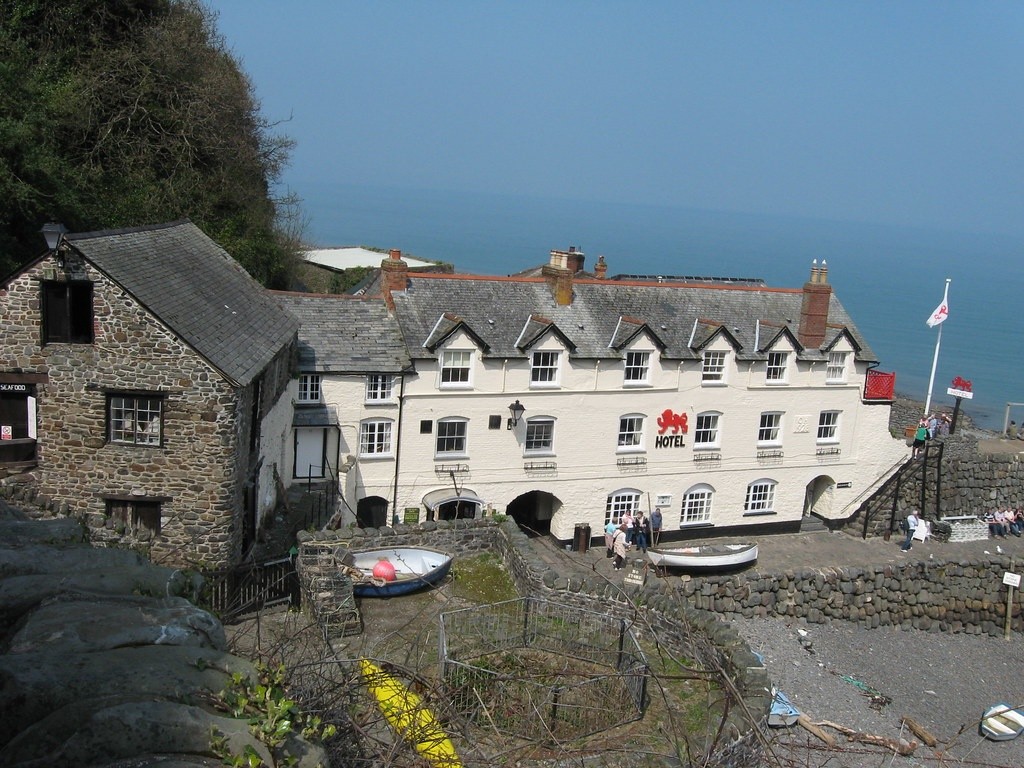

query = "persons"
[900, 509, 920, 553]
[604, 507, 662, 572]
[1008, 420, 1024, 441]
[983, 502, 1024, 540]
[913, 411, 952, 460]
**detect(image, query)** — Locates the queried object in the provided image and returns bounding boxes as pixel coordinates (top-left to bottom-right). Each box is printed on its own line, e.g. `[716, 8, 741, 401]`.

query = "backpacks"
[903, 519, 909, 530]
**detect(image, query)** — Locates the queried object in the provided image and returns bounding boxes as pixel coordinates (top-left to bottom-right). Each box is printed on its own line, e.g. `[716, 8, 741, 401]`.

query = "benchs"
[710, 545, 732, 552]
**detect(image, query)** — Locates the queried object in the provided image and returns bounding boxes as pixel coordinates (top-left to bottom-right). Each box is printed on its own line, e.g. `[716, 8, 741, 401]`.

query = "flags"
[926, 298, 949, 328]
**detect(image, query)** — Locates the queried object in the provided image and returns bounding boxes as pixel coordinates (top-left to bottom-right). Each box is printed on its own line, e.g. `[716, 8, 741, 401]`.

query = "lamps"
[507, 400, 525, 430]
[38, 216, 68, 267]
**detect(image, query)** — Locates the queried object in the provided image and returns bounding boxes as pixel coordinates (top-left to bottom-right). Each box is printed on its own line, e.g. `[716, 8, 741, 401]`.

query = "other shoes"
[613, 561, 616, 565]
[614, 568, 621, 571]
[994, 534, 1000, 540]
[1004, 535, 1009, 540]
[636, 547, 640, 550]
[900, 549, 907, 552]
[607, 557, 613, 559]
[642, 549, 646, 553]
[1016, 531, 1022, 537]
[907, 547, 912, 551]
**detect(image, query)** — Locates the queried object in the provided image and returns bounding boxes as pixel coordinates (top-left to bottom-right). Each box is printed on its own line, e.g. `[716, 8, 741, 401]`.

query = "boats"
[751, 650, 800, 726]
[981, 702, 1024, 741]
[646, 542, 758, 566]
[359, 656, 463, 768]
[350, 546, 454, 598]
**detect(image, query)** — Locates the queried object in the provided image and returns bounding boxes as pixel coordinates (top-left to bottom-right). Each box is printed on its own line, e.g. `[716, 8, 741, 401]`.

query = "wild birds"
[996, 545, 1006, 555]
[983, 550, 990, 556]
[929, 553, 933, 561]
